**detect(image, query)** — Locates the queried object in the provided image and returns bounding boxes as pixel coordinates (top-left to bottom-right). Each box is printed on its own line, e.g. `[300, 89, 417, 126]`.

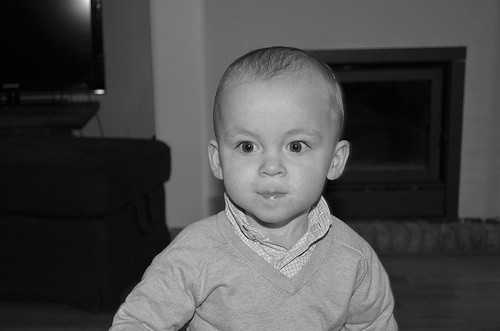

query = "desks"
[0, 101, 100, 130]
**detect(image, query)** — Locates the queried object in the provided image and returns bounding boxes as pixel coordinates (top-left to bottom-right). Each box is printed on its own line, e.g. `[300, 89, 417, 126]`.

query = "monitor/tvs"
[0, 0, 105, 96]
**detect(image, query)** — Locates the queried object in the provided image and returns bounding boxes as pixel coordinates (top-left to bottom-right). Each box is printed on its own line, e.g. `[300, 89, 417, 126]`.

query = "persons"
[108, 45, 399, 331]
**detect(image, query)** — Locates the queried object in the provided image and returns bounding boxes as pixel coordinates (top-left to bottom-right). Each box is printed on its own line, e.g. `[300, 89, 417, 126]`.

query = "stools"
[0, 130, 172, 313]
